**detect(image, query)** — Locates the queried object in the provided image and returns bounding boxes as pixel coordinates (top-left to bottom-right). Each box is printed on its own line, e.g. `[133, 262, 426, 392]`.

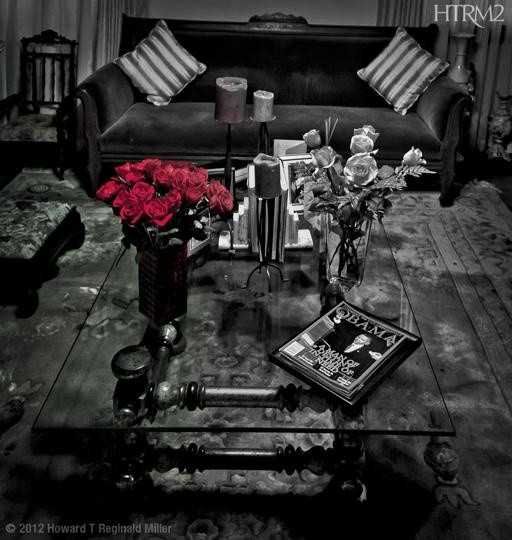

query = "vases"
[449, 32, 477, 120]
[486, 89, 512, 148]
[326, 212, 373, 290]
[486, 140, 511, 173]
[138, 242, 189, 326]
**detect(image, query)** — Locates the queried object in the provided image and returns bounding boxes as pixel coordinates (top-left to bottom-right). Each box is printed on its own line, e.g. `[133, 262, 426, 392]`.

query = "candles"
[214, 77, 248, 124]
[250, 90, 277, 123]
[253, 152, 282, 201]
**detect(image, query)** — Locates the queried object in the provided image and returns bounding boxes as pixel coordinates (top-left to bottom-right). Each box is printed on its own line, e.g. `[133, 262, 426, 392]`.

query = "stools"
[0, 198, 85, 319]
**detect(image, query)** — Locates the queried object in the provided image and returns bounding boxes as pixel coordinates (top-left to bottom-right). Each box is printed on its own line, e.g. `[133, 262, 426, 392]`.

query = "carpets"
[0, 167, 511, 540]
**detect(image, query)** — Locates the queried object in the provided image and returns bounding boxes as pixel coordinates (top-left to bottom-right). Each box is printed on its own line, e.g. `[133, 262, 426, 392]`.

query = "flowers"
[288, 119, 437, 226]
[96, 156, 235, 253]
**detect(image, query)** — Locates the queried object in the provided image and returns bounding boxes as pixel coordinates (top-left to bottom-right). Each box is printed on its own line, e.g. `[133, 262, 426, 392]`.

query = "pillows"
[357, 25, 450, 117]
[112, 17, 206, 108]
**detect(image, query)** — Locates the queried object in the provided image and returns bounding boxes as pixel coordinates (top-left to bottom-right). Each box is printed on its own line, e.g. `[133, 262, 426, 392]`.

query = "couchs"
[65, 13, 473, 207]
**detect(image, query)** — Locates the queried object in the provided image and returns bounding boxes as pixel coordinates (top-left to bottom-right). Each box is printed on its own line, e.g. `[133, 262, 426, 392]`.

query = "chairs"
[0, 30, 80, 181]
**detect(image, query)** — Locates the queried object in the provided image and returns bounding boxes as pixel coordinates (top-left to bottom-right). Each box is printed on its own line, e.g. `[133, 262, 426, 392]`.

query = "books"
[268, 300, 422, 413]
